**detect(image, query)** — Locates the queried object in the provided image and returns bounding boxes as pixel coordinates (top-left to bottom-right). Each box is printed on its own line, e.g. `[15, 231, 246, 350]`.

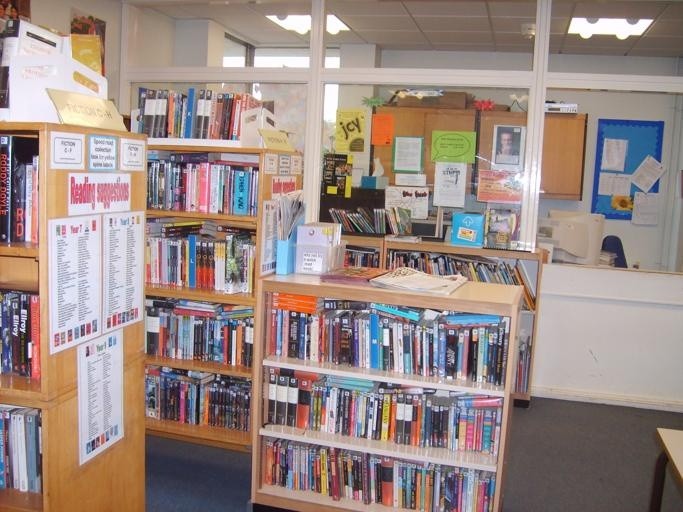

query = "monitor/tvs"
[547, 210, 607, 266]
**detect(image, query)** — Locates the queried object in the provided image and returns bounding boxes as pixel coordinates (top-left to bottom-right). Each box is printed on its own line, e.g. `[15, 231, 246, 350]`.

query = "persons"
[494, 131, 521, 157]
[5, 4, 21, 37]
[0, 2, 9, 37]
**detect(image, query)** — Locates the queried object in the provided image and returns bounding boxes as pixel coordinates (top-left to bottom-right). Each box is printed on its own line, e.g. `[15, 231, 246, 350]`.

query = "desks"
[649, 427, 682, 511]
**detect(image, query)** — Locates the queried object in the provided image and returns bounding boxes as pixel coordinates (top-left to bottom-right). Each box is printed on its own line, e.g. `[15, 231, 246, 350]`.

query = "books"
[514, 336, 533, 393]
[274, 186, 347, 279]
[0, 130, 41, 499]
[329, 204, 517, 250]
[131, 85, 273, 217]
[261, 364, 503, 456]
[344, 245, 532, 310]
[145, 215, 255, 368]
[261, 435, 496, 512]
[272, 291, 511, 388]
[144, 364, 253, 432]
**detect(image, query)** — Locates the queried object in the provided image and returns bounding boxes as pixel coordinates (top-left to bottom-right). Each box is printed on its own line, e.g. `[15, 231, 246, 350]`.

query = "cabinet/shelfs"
[0, 118, 551, 511]
[373, 105, 588, 200]
